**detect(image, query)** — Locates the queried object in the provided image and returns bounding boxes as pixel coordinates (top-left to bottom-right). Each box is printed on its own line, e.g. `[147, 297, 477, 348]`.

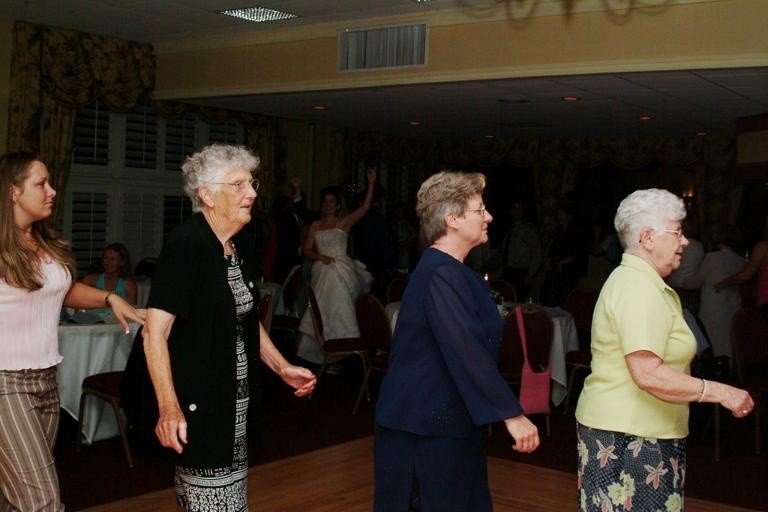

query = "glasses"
[464, 207, 485, 215]
[212, 179, 259, 191]
[662, 229, 683, 239]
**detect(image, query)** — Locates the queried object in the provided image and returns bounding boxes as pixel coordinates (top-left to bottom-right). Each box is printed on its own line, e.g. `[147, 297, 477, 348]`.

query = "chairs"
[54, 247, 768, 472]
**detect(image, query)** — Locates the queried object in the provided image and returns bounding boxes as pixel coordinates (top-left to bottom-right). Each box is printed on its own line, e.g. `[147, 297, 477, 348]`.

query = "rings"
[742, 410, 747, 415]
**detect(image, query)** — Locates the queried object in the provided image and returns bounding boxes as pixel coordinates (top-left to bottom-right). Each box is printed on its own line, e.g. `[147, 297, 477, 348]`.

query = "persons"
[392, 146, 767, 360]
[2, 148, 146, 511]
[142, 146, 316, 511]
[576, 187, 754, 510]
[374, 172, 541, 510]
[299, 166, 377, 373]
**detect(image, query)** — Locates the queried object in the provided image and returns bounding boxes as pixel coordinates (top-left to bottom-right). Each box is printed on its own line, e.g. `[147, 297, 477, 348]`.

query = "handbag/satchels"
[518, 364, 551, 415]
